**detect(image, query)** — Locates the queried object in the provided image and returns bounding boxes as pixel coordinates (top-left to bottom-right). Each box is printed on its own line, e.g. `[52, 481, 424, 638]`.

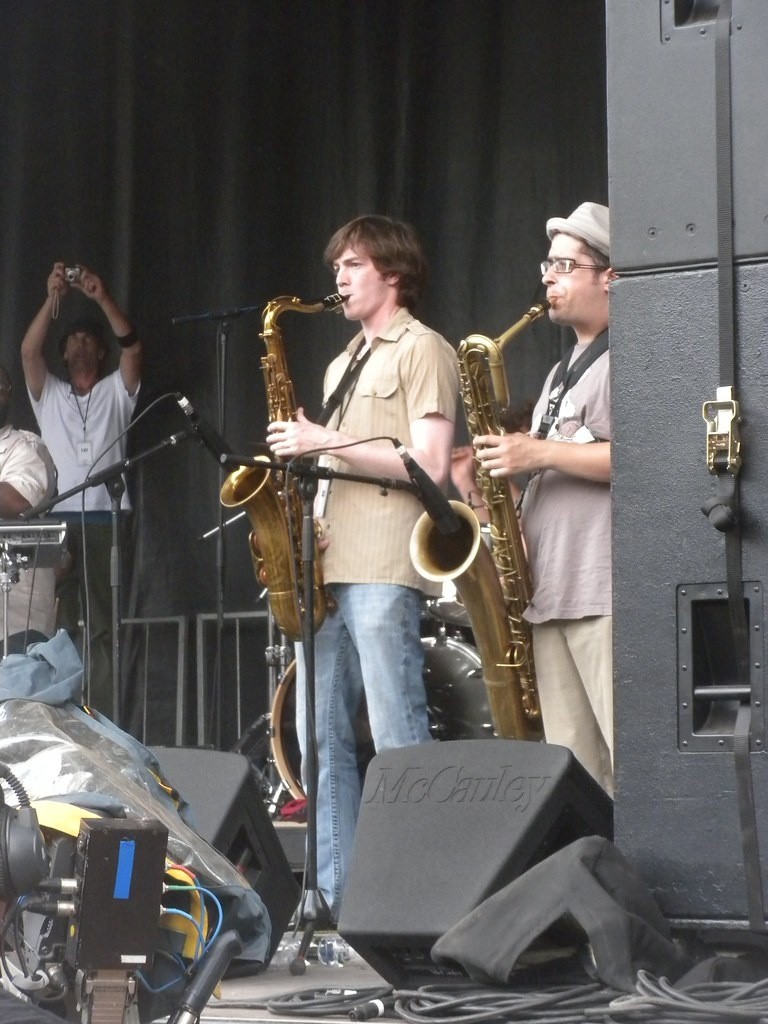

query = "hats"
[546, 201, 610, 258]
[58, 318, 110, 364]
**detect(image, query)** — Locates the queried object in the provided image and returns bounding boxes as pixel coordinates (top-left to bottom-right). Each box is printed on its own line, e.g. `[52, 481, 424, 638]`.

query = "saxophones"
[406, 295, 552, 743]
[213, 290, 347, 643]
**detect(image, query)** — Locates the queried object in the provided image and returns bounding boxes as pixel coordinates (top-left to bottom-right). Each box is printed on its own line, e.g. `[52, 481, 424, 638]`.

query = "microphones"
[391, 438, 461, 536]
[177, 394, 240, 474]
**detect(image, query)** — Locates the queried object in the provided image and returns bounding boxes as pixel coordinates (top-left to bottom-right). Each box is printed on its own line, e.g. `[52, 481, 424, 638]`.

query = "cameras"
[64, 266, 81, 282]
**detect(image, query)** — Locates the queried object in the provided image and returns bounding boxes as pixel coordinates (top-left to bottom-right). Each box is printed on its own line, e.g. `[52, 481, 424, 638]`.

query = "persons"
[448, 203, 613, 803]
[0, 361, 74, 660]
[264, 216, 462, 955]
[19, 261, 142, 722]
[450, 397, 535, 558]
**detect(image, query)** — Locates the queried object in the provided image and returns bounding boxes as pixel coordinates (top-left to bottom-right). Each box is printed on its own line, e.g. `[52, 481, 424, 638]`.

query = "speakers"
[336, 0, 768, 989]
[0, 521, 68, 642]
[20, 744, 303, 983]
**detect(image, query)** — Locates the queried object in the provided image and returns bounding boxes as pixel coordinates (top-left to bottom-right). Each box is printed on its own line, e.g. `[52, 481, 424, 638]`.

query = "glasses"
[540, 259, 609, 275]
